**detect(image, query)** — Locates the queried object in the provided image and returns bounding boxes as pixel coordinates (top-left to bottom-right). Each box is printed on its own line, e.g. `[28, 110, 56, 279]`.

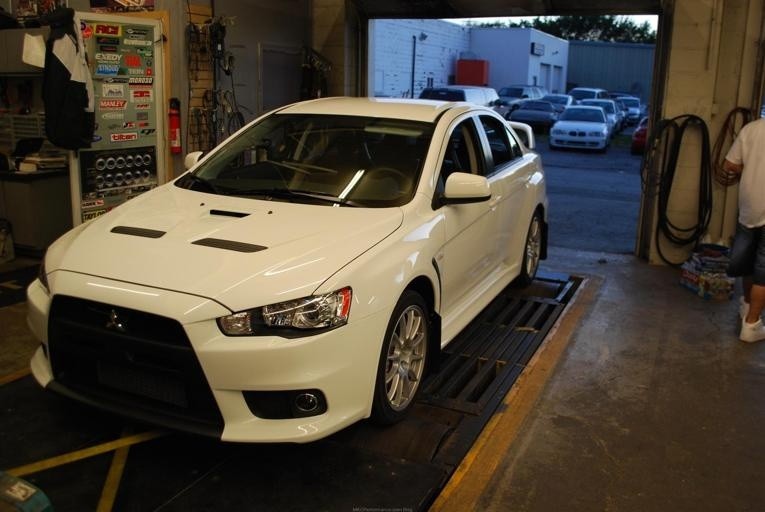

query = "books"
[17, 154, 68, 171]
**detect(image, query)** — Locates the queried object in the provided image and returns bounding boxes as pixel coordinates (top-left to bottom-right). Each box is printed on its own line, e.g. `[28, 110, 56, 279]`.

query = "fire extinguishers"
[168, 97, 183, 155]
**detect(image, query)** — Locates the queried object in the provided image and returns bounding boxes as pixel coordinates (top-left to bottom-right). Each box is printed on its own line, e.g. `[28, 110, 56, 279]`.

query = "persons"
[723, 117, 765, 343]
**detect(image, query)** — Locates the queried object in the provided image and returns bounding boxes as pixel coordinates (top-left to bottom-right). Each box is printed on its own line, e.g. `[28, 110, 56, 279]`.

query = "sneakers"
[738, 295, 765, 342]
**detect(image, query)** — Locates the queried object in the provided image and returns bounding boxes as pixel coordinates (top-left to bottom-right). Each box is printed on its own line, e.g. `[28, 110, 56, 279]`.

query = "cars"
[20, 94, 551, 449]
[418, 80, 649, 157]
